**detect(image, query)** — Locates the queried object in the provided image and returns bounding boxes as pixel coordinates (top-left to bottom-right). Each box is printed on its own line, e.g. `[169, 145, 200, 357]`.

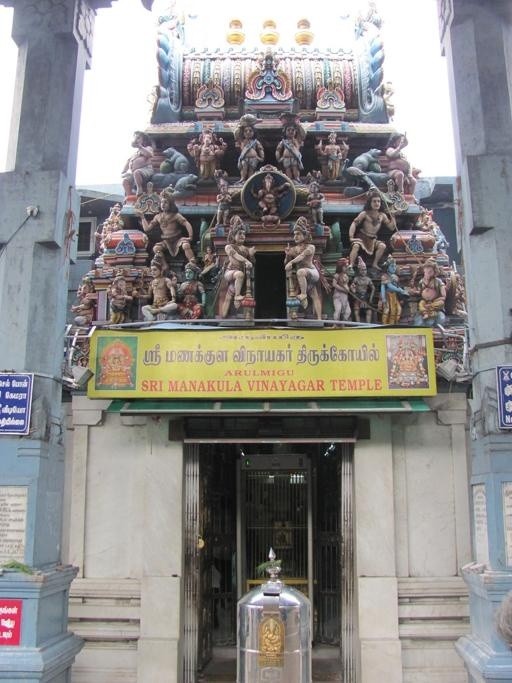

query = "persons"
[273, 117, 306, 183]
[383, 129, 420, 205]
[317, 129, 349, 176]
[233, 120, 265, 183]
[207, 537, 341, 646]
[72, 181, 446, 326]
[120, 129, 156, 199]
